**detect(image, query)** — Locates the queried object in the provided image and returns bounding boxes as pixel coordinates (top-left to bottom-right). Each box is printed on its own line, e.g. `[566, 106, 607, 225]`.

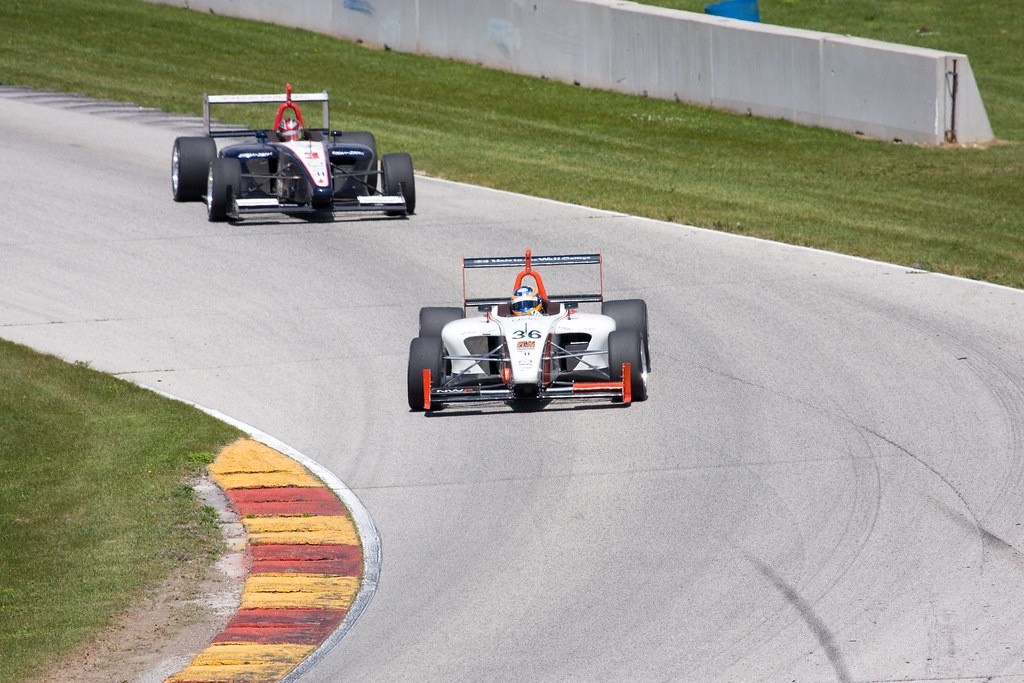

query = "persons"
[275, 118, 305, 142]
[509, 285, 544, 316]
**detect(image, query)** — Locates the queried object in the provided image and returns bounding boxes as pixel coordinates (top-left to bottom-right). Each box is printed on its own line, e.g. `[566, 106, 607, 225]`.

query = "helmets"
[511, 286, 543, 315]
[277, 118, 304, 142]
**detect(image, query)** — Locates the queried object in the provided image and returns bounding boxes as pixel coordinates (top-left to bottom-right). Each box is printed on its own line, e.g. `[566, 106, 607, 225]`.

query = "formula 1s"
[403, 250, 652, 412]
[170, 83, 417, 224]
